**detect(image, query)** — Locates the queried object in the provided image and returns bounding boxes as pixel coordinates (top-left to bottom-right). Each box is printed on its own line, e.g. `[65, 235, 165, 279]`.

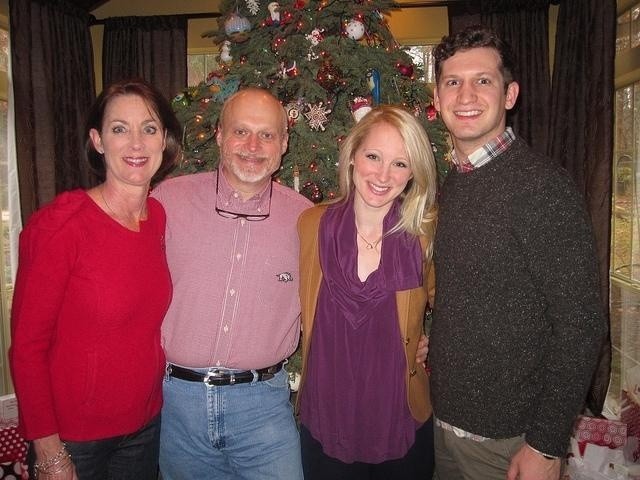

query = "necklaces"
[358, 230, 377, 250]
[100, 184, 138, 231]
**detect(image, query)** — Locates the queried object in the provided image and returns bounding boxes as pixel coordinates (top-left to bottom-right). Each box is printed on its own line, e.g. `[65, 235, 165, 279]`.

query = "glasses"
[214, 168, 274, 221]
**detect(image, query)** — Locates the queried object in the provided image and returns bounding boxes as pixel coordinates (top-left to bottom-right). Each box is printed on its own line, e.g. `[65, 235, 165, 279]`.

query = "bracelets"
[31, 442, 73, 478]
[527, 443, 559, 461]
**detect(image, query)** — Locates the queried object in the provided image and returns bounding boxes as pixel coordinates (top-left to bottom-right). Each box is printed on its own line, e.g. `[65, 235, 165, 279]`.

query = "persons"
[7, 78, 185, 480]
[148, 88, 428, 480]
[295, 108, 439, 480]
[427, 24, 608, 478]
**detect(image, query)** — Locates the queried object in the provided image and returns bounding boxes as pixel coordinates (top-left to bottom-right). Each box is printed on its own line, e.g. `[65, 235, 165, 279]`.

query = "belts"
[167, 361, 284, 387]
